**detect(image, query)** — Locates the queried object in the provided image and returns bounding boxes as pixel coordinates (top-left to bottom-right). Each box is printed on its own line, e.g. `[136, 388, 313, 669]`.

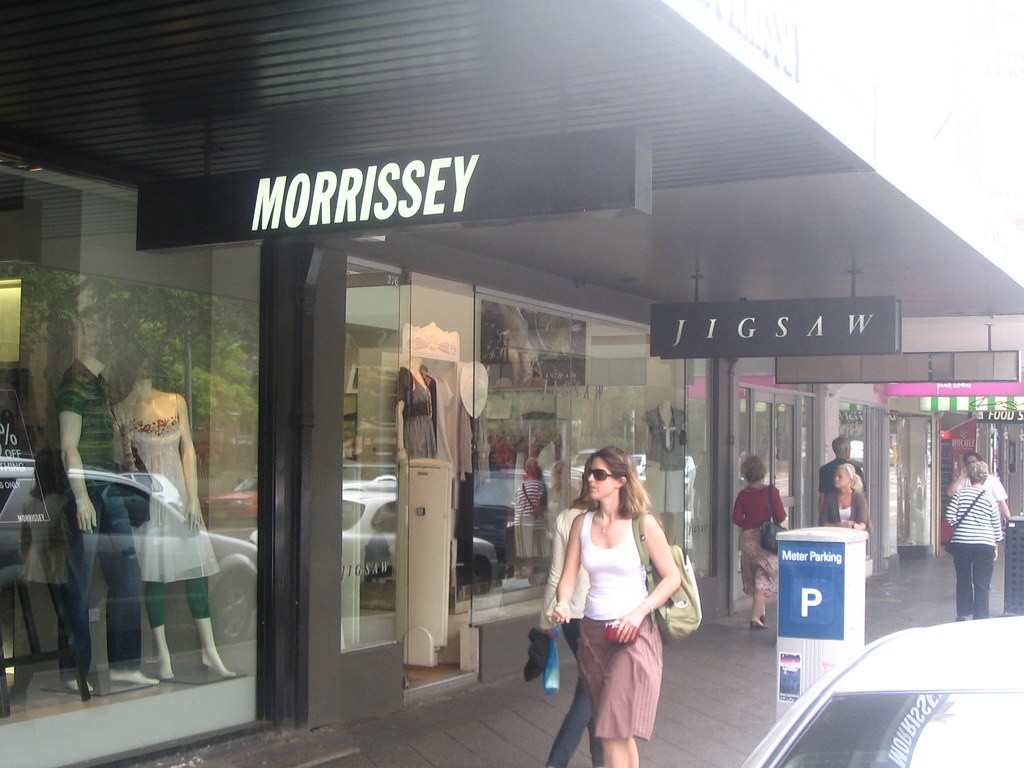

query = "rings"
[557, 617, 560, 622]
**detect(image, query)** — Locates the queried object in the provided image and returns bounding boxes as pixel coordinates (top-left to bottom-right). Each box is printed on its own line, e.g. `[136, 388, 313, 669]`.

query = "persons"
[818, 463, 869, 530]
[946, 452, 1011, 518]
[534, 472, 604, 768]
[395, 355, 437, 461]
[732, 456, 787, 629]
[553, 446, 681, 768]
[644, 399, 687, 514]
[945, 461, 1003, 622]
[514, 456, 579, 575]
[113, 378, 236, 679]
[818, 437, 867, 513]
[56, 354, 160, 691]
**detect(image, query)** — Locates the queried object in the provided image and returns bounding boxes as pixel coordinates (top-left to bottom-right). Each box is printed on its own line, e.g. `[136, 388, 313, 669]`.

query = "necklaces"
[657, 405, 675, 453]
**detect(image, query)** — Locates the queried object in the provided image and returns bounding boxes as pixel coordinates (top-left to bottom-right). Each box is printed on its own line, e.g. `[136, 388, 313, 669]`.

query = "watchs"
[643, 599, 654, 612]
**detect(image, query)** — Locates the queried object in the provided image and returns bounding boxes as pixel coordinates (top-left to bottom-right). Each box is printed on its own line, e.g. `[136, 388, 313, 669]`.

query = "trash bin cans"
[1001, 515, 1024, 617]
[774, 525, 869, 723]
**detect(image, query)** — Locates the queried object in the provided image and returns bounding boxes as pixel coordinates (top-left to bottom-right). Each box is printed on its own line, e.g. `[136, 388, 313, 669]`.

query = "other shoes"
[954, 616, 965, 622]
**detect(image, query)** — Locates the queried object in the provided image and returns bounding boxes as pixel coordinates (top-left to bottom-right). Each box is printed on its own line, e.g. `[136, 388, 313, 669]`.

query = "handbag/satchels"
[541, 625, 561, 695]
[945, 535, 955, 557]
[758, 484, 788, 553]
[533, 507, 543, 520]
[853, 491, 875, 534]
[632, 510, 702, 644]
[523, 628, 551, 682]
[112, 470, 152, 527]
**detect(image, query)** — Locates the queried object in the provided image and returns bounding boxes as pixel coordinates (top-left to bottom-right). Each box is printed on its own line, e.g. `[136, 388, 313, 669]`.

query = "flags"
[951, 418, 976, 457]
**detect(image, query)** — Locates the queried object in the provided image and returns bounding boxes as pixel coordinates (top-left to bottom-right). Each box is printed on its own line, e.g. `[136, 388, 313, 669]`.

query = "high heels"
[751, 621, 768, 630]
[760, 617, 766, 622]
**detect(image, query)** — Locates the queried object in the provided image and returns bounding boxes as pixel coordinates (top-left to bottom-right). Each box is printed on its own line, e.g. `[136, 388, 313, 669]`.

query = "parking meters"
[776, 527, 869, 724]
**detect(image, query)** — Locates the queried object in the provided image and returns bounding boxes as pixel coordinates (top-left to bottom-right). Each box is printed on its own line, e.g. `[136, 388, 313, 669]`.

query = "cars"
[0, 451, 789, 642]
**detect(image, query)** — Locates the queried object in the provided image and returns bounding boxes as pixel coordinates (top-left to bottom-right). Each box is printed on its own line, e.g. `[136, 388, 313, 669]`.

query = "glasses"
[585, 467, 616, 481]
